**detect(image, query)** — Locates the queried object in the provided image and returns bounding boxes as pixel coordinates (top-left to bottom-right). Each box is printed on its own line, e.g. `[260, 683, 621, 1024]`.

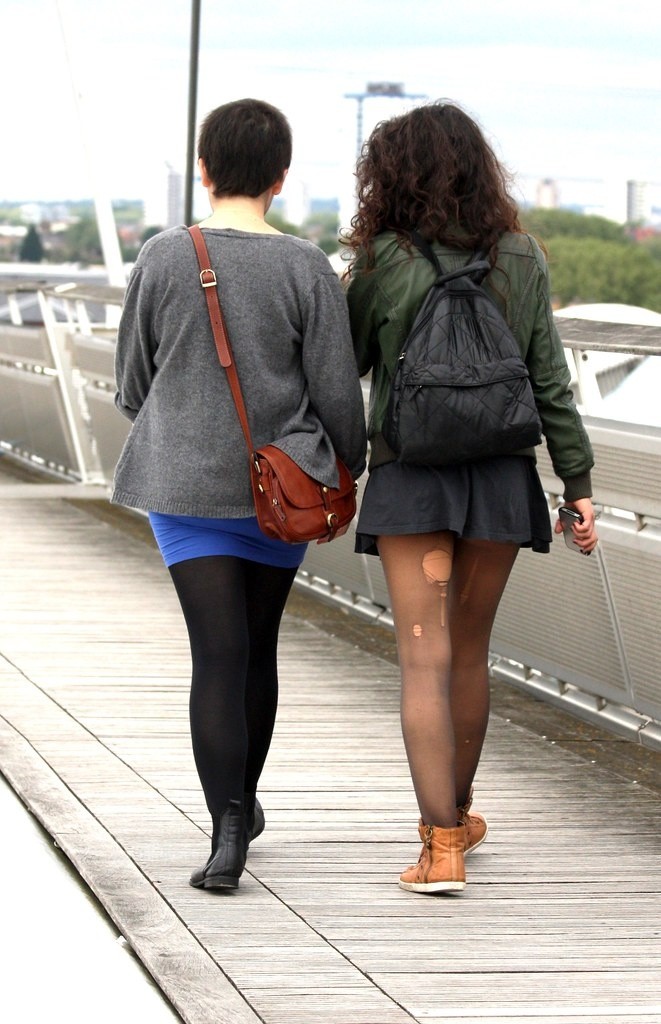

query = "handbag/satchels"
[250, 441, 358, 546]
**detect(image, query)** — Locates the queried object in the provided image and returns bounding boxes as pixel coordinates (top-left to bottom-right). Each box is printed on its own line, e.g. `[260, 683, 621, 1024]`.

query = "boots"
[397, 784, 488, 892]
[188, 794, 264, 889]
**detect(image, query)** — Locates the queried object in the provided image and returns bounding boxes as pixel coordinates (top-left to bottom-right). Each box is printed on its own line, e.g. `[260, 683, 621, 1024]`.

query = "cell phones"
[558, 506, 592, 556]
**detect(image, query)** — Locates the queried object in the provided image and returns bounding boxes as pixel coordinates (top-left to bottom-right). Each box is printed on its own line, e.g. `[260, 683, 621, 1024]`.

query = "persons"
[109, 97, 369, 893]
[16, 223, 46, 263]
[336, 101, 601, 896]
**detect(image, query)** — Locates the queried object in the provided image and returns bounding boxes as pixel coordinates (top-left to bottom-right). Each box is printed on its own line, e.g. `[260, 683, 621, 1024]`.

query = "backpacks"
[381, 224, 542, 460]
